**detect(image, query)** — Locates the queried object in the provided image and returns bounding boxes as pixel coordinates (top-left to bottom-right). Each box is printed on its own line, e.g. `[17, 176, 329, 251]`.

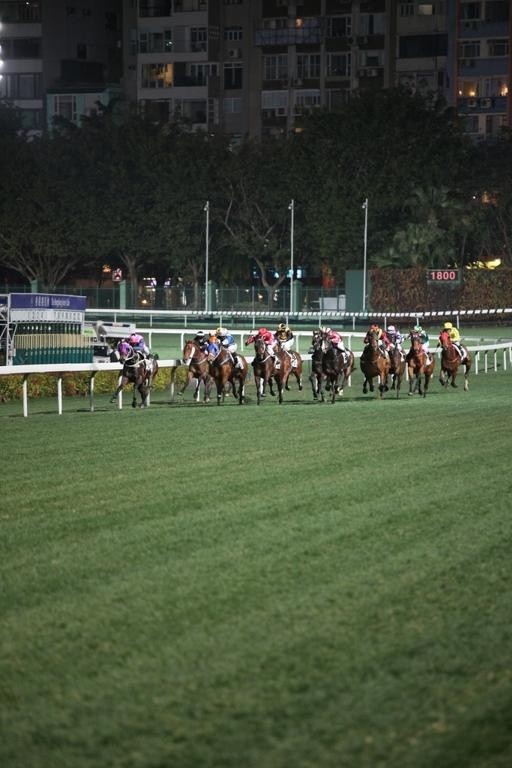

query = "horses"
[108, 344, 159, 410]
[250, 336, 281, 405]
[320, 333, 355, 405]
[308, 329, 335, 401]
[359, 329, 390, 394]
[438, 330, 473, 392]
[384, 334, 408, 399]
[268, 338, 303, 404]
[177, 339, 248, 403]
[407, 329, 436, 399]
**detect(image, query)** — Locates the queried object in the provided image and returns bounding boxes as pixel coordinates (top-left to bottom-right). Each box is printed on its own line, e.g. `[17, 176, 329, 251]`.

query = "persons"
[192, 323, 467, 378]
[115, 334, 151, 376]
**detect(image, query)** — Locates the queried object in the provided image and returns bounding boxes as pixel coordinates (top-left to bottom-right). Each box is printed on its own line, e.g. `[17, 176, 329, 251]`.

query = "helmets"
[444, 323, 452, 329]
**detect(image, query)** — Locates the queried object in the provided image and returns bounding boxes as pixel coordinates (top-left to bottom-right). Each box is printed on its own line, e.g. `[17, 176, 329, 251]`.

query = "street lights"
[202, 201, 212, 311]
[287, 198, 299, 315]
[360, 198, 371, 315]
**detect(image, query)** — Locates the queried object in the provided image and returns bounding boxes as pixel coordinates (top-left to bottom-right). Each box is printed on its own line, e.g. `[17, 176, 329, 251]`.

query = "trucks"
[88, 320, 138, 360]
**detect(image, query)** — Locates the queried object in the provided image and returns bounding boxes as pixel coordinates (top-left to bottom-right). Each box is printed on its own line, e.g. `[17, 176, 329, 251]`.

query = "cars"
[90, 342, 113, 363]
